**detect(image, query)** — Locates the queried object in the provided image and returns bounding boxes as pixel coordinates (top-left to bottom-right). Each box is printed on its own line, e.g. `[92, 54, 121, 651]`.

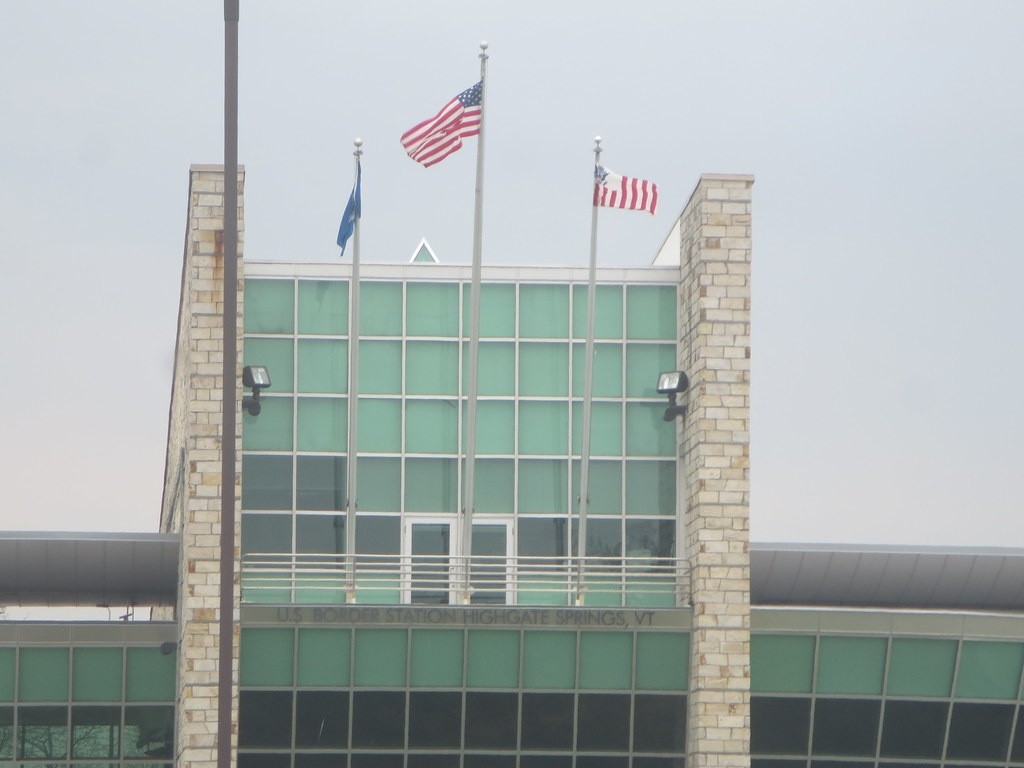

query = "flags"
[336, 160, 361, 257]
[592, 164, 657, 216]
[399, 81, 483, 168]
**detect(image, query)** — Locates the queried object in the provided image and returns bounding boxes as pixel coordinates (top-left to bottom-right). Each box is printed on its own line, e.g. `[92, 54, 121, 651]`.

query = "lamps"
[160, 641, 177, 654]
[241, 366, 272, 414]
[656, 371, 690, 422]
